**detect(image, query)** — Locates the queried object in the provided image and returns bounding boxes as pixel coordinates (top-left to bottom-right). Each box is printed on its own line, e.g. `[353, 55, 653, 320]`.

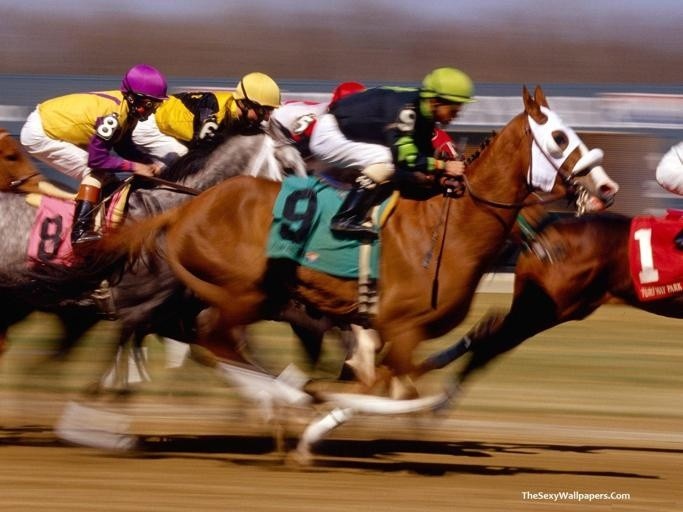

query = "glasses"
[240, 79, 274, 115]
[123, 75, 161, 111]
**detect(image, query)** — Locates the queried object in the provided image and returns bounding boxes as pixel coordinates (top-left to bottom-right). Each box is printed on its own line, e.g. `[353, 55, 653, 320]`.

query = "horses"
[0, 83, 621, 468]
[409, 208, 683, 418]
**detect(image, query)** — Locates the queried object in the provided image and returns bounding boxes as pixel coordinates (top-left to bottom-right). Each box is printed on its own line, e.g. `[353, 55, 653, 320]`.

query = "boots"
[330, 173, 379, 235]
[71, 200, 101, 244]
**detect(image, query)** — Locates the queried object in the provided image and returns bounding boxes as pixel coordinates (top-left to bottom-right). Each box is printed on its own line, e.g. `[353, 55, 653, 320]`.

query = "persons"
[116, 71, 281, 172]
[263, 78, 366, 151]
[15, 64, 167, 246]
[304, 66, 476, 243]
[654, 137, 683, 253]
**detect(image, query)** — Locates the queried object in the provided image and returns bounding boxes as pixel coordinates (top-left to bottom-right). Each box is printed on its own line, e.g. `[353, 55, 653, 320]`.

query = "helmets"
[231, 72, 281, 107]
[333, 82, 366, 103]
[419, 67, 479, 103]
[121, 63, 169, 100]
